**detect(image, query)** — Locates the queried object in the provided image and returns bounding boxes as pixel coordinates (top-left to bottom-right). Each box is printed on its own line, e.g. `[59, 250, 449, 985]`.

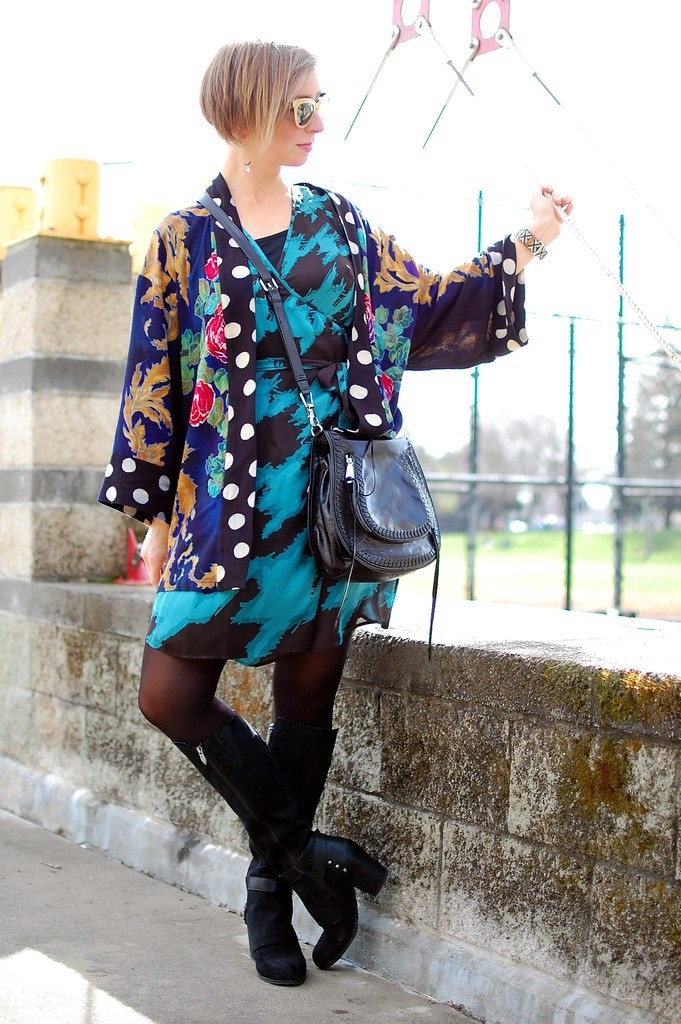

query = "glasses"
[287, 91, 325, 129]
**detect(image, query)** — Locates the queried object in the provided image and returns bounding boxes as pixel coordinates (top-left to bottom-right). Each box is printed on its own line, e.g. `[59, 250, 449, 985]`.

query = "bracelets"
[514, 228, 548, 260]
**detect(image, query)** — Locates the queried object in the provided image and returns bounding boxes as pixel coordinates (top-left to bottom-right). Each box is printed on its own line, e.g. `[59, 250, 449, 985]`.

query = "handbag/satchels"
[306, 429, 441, 582]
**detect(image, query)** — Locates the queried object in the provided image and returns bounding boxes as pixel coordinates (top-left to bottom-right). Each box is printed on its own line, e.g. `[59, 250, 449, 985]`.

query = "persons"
[98, 38, 575, 987]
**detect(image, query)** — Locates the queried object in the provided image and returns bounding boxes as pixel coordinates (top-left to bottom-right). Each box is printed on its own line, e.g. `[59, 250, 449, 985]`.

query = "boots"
[244, 722, 340, 985]
[174, 712, 387, 970]
